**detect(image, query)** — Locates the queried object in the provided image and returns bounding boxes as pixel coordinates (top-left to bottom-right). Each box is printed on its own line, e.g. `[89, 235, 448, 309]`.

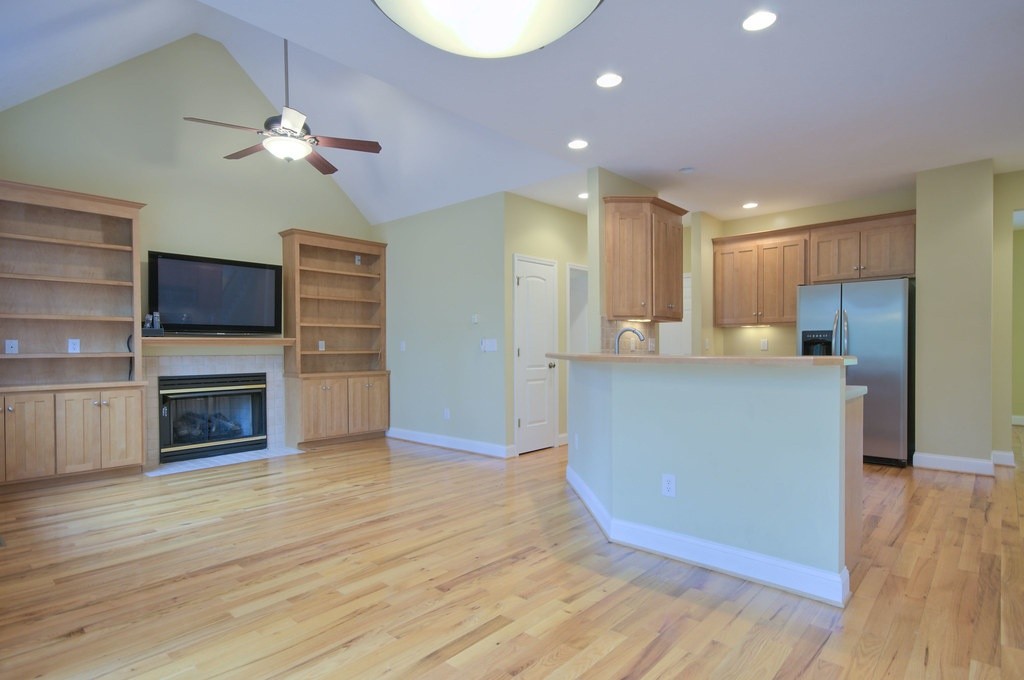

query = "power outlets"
[4, 339, 19, 353]
[68, 339, 80, 353]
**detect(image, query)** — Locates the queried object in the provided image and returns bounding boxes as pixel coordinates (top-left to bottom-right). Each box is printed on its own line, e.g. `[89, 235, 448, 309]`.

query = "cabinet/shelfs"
[278, 228, 391, 448]
[713, 229, 808, 328]
[0, 179, 149, 495]
[603, 197, 689, 321]
[809, 214, 916, 285]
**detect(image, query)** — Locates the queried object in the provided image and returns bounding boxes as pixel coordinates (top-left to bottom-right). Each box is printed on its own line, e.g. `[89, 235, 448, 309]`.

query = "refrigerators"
[796, 277, 914, 468]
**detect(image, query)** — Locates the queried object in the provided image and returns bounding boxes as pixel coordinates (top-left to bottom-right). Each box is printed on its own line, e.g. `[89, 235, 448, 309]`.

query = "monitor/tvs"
[148, 251, 282, 335]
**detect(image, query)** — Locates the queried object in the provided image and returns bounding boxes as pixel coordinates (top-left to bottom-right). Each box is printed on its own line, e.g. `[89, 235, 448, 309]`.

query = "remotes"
[153, 312, 160, 328]
[144, 314, 152, 328]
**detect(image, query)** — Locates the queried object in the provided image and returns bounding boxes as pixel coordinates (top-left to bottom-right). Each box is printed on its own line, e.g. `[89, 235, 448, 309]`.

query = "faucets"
[614, 326, 645, 355]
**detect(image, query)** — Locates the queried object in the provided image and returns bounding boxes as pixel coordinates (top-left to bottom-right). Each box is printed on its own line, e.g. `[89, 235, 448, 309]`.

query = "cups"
[153, 312, 161, 329]
[144, 314, 153, 328]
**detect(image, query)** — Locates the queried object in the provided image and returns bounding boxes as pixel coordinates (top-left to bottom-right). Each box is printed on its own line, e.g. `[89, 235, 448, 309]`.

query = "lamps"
[372, 0, 605, 58]
[262, 132, 314, 163]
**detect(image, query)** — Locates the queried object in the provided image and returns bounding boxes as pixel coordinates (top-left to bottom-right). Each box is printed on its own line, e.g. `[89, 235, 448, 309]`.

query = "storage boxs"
[142, 321, 164, 337]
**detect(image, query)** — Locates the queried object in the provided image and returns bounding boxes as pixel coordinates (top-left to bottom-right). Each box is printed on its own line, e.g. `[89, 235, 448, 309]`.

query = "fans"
[182, 39, 383, 175]
